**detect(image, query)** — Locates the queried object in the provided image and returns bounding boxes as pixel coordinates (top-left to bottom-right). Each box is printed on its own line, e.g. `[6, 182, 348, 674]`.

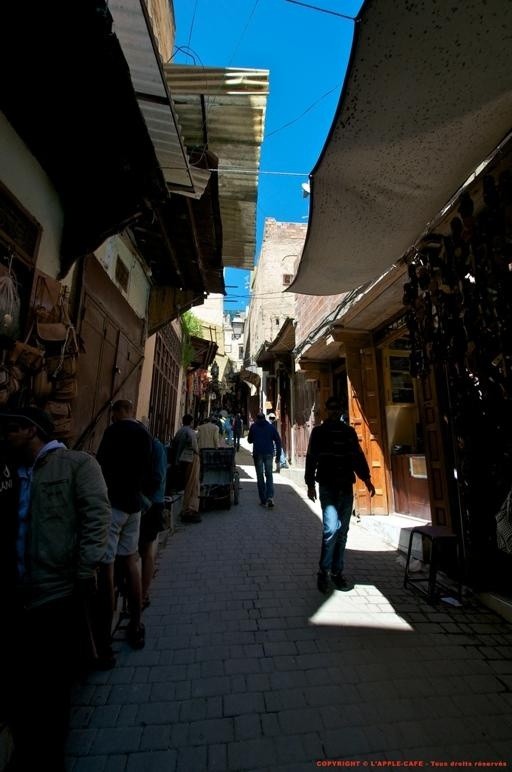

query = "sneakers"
[266, 498, 274, 508]
[317, 572, 353, 591]
[125, 623, 146, 651]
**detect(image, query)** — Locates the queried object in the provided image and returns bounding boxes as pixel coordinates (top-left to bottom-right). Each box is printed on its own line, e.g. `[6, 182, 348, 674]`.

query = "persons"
[2, 404, 113, 771]
[142, 436, 168, 610]
[170, 408, 253, 523]
[304, 395, 375, 595]
[248, 412, 281, 508]
[94, 396, 145, 672]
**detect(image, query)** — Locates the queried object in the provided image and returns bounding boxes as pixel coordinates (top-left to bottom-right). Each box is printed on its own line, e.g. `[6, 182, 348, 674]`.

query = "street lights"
[207, 360, 219, 417]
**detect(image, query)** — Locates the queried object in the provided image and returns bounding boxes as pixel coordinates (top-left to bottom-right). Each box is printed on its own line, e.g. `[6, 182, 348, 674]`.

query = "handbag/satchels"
[0, 306, 78, 439]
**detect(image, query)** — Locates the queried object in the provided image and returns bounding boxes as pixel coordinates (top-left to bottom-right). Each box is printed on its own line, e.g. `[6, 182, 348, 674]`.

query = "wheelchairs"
[198, 446, 244, 511]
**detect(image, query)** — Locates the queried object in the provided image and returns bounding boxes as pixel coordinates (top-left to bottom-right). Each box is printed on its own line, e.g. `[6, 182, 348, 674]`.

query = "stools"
[404, 526, 465, 606]
